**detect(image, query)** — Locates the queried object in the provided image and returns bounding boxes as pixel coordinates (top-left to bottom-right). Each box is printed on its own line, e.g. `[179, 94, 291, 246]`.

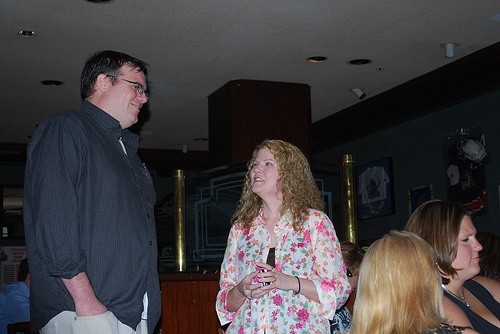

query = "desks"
[156, 273, 357, 334]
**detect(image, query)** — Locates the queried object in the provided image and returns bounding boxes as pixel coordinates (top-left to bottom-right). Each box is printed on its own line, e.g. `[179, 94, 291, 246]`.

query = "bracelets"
[292, 276, 300, 294]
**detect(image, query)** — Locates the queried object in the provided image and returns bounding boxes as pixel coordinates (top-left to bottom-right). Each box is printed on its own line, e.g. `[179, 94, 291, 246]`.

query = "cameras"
[259, 269, 268, 287]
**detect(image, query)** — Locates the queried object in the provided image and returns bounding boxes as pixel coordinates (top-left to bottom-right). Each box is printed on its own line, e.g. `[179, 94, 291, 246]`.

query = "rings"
[271, 266, 274, 273]
[272, 277, 276, 283]
[251, 290, 254, 299]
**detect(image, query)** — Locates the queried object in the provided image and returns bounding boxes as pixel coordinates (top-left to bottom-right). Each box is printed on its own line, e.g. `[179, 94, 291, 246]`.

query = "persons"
[348, 229, 462, 334]
[23, 50, 161, 334]
[0, 258, 30, 334]
[215, 138, 352, 334]
[343, 248, 366, 290]
[403, 200, 500, 334]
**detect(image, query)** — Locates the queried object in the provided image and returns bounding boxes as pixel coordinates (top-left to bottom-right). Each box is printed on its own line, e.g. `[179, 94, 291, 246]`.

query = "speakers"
[207, 80, 312, 166]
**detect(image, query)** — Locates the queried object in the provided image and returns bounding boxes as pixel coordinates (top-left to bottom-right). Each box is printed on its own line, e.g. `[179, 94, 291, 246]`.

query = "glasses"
[106, 75, 149, 99]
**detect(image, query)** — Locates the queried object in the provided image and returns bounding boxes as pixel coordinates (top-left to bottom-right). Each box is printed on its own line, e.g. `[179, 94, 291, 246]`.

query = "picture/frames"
[409, 183, 434, 218]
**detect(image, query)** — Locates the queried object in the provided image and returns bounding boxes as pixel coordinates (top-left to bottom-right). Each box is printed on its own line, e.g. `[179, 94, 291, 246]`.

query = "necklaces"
[442, 281, 470, 307]
